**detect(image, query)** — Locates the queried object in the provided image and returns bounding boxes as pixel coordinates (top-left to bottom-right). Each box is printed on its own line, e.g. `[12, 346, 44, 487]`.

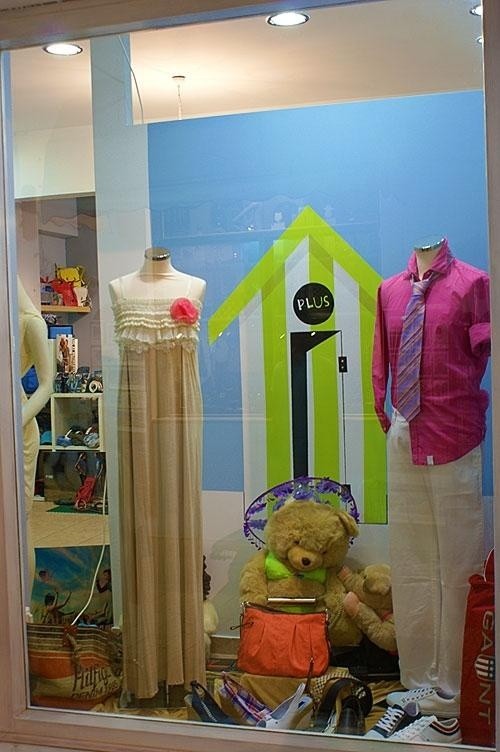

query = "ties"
[396, 247, 456, 423]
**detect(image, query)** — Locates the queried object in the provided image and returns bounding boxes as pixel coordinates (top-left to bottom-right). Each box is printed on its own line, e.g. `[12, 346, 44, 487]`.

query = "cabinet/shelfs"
[34, 299, 109, 514]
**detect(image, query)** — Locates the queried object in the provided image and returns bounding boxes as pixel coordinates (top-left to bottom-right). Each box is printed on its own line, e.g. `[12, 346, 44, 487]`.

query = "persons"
[370, 238, 491, 695]
[16, 275, 55, 626]
[109, 249, 208, 696]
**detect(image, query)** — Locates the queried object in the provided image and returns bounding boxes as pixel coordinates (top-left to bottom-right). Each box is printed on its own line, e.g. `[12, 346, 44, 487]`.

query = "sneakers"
[364, 686, 463, 745]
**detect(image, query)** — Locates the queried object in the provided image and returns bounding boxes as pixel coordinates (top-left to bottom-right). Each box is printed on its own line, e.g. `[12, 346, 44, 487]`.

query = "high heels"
[183, 671, 314, 731]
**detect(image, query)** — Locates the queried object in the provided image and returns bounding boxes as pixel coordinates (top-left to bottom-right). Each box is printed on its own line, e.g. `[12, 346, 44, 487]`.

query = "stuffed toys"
[336, 563, 399, 654]
[241, 493, 367, 649]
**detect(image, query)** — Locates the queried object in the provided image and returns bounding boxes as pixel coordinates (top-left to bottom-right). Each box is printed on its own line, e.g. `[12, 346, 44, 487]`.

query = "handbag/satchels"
[337, 695, 365, 736]
[237, 606, 332, 678]
[27, 621, 124, 711]
